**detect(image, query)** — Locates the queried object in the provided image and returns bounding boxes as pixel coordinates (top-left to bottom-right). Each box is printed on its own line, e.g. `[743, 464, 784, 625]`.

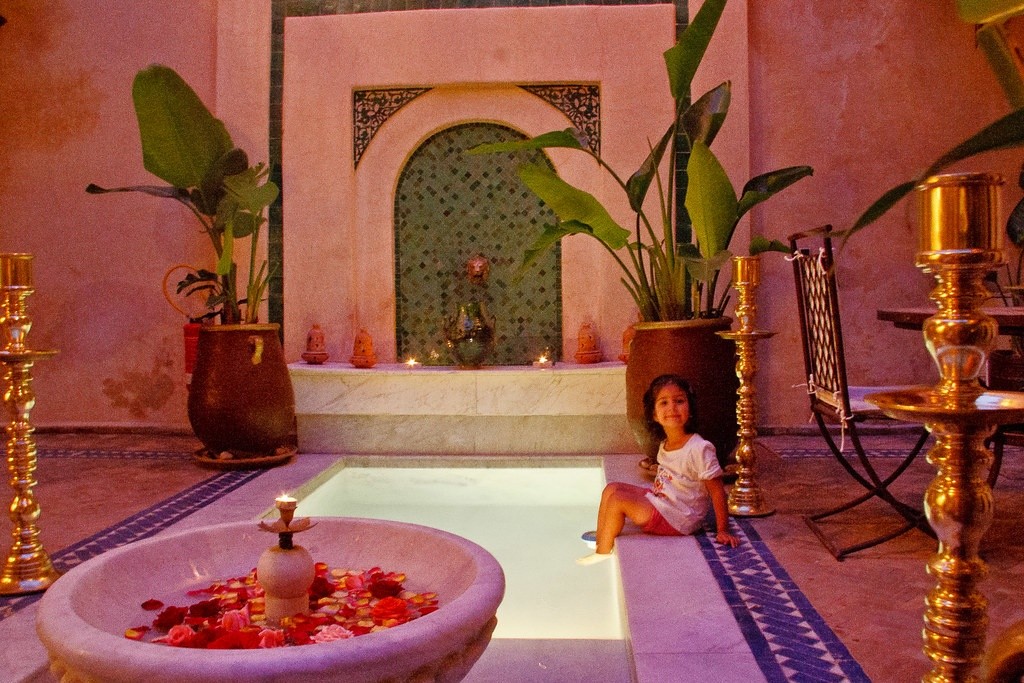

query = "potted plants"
[83, 62, 302, 461]
[463, 0, 814, 478]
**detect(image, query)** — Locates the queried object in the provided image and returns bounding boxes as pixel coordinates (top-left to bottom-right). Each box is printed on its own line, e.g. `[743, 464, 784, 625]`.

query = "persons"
[596, 375, 740, 554]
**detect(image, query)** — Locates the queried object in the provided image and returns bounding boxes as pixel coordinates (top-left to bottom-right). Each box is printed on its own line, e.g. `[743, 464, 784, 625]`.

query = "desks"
[872, 307, 1023, 337]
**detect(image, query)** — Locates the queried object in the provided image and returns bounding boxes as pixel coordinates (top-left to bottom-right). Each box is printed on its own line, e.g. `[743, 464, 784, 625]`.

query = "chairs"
[785, 221, 1006, 564]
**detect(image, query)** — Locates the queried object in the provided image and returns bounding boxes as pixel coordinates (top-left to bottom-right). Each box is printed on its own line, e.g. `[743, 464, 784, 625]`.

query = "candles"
[276, 494, 297, 509]
[402, 358, 422, 370]
[533, 355, 552, 368]
